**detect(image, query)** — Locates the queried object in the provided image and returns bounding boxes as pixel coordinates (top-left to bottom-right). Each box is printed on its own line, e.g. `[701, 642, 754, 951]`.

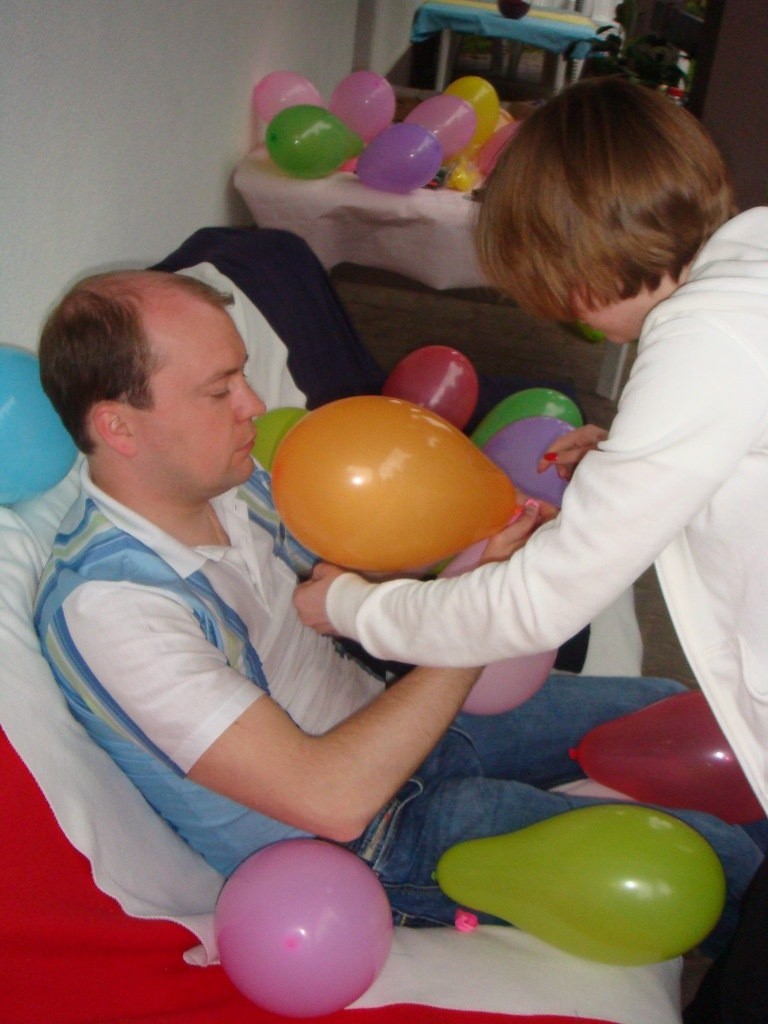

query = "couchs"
[0, 223, 690, 1024]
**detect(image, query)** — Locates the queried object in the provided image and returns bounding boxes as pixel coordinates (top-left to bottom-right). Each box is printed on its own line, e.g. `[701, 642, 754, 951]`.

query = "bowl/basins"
[492, 0, 532, 20]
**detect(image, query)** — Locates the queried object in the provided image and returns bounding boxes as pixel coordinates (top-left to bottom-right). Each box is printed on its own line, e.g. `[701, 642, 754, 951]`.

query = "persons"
[294, 80, 768, 1024]
[34, 270, 768, 1009]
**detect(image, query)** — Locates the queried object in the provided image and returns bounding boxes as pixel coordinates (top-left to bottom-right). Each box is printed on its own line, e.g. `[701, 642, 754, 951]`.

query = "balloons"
[216, 67, 768, 1016]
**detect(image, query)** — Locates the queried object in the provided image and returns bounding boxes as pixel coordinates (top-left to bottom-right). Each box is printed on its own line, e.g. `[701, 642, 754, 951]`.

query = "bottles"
[668, 85, 686, 106]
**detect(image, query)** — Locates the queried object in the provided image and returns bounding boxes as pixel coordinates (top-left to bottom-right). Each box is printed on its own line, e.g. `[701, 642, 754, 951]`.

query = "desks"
[236, 143, 633, 403]
[421, 0, 606, 98]
